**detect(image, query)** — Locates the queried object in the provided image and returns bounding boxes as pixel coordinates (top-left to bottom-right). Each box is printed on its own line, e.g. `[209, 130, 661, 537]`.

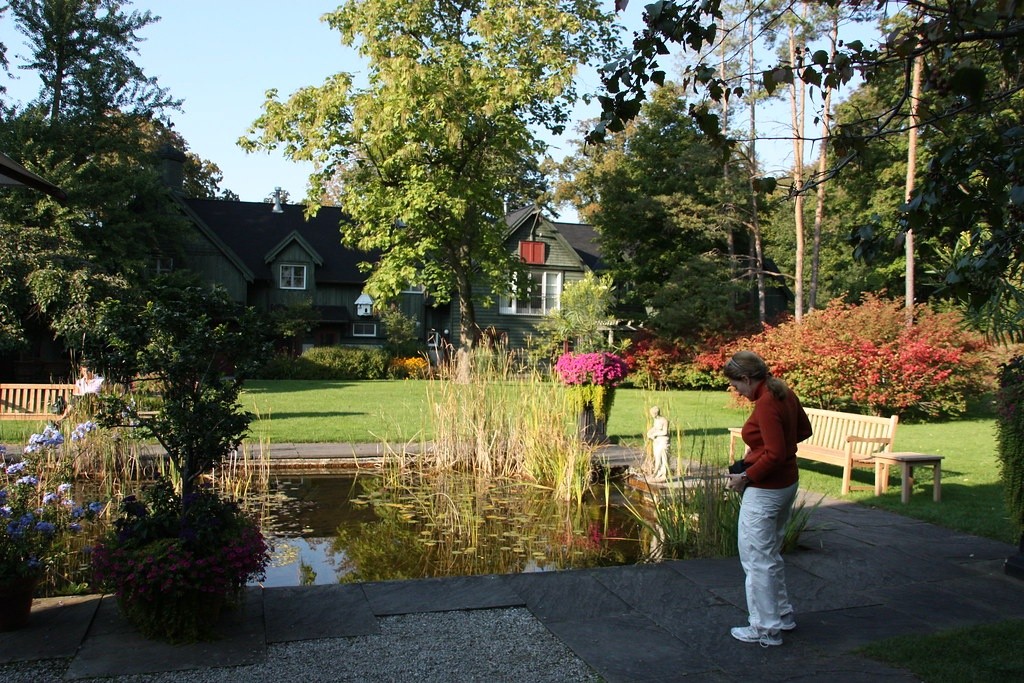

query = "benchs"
[0, 384, 123, 421]
[728, 406, 899, 496]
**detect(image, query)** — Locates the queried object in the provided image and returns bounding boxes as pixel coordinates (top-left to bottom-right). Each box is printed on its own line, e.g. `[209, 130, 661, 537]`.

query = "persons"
[647, 406, 670, 482]
[56, 359, 106, 423]
[723, 350, 812, 645]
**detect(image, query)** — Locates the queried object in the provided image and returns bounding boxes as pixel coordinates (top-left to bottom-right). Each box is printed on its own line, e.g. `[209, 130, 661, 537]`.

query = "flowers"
[0, 419, 104, 590]
[556, 352, 630, 430]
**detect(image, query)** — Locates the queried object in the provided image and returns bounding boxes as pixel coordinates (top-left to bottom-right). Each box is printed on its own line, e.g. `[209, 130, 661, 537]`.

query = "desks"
[872, 452, 944, 505]
[133, 411, 160, 424]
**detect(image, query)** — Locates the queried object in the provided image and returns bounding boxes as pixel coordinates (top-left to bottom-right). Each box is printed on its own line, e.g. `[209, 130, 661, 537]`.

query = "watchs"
[740, 472, 749, 484]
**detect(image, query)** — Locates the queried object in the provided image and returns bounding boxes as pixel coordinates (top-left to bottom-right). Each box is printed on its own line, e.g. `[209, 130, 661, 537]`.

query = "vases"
[580, 401, 606, 445]
[0, 564, 40, 630]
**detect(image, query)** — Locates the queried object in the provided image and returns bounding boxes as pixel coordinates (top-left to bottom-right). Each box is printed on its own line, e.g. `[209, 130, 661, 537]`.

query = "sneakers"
[778, 610, 796, 630]
[731, 626, 783, 647]
